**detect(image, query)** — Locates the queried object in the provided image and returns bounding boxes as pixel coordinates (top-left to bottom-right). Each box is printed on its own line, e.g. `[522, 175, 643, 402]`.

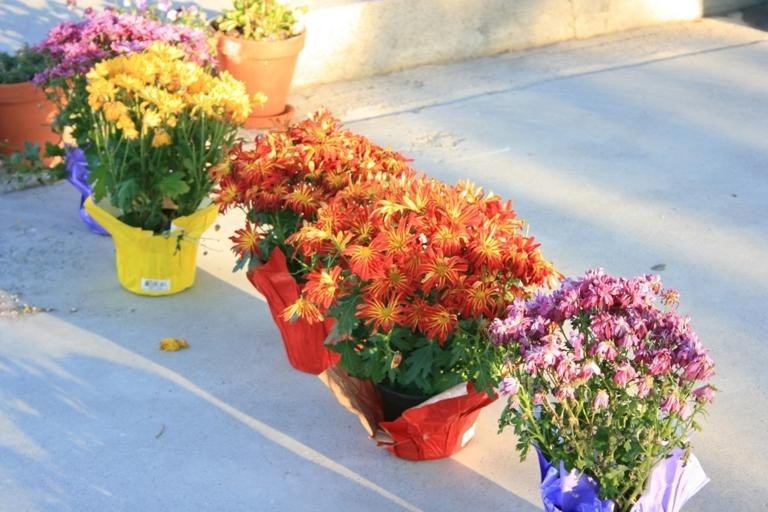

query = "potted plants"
[0, 43, 67, 178]
[206, 2, 312, 120]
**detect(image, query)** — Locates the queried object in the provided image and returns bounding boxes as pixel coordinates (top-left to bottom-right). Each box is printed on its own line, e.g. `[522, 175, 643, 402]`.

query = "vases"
[104, 204, 207, 300]
[362, 376, 463, 463]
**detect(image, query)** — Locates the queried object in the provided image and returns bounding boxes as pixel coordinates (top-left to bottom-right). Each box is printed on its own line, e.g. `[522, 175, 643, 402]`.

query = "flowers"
[31, 1, 225, 102]
[481, 262, 718, 500]
[204, 100, 420, 286]
[274, 171, 562, 403]
[79, 42, 268, 221]
